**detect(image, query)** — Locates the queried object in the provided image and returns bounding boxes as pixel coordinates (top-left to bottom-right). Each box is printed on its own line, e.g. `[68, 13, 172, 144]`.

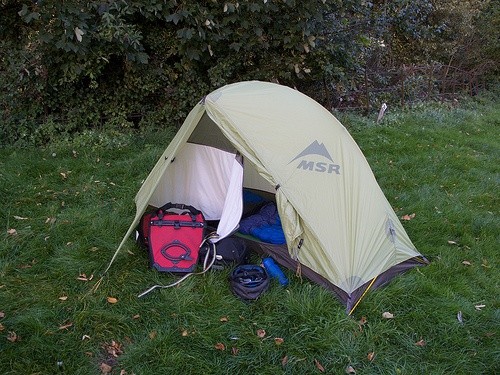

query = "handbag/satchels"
[149, 202, 207, 273]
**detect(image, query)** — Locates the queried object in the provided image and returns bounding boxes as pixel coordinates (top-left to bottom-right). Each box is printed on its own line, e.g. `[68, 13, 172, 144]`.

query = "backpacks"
[137, 208, 156, 251]
[199, 236, 248, 265]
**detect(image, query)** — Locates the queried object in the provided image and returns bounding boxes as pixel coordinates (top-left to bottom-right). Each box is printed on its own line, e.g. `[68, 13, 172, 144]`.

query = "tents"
[104, 80, 429, 319]
